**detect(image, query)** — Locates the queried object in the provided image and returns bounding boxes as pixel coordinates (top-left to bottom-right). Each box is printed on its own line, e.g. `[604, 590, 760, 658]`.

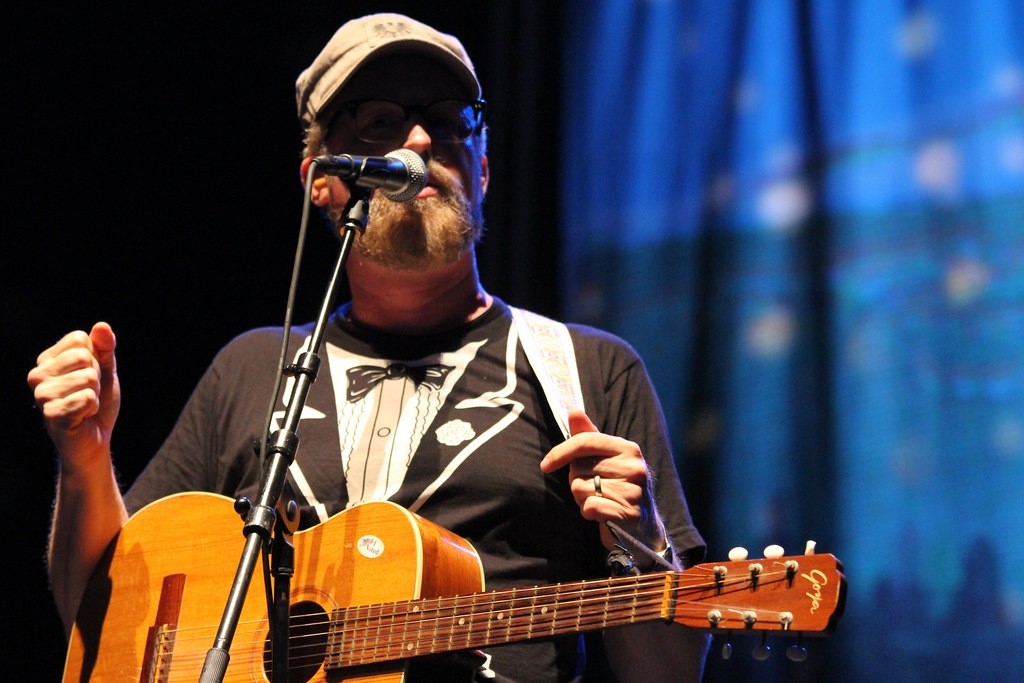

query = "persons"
[27, 5, 717, 683]
[594, 475, 603, 497]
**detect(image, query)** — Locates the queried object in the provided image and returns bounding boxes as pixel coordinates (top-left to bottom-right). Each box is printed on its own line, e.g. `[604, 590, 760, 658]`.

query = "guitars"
[61, 490, 846, 683]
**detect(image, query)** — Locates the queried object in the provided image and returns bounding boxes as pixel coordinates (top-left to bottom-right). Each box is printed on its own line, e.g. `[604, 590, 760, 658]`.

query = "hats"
[295, 12, 482, 124]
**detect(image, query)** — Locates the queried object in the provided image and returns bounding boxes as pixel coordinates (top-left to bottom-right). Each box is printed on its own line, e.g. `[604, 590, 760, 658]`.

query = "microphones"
[311, 149, 430, 203]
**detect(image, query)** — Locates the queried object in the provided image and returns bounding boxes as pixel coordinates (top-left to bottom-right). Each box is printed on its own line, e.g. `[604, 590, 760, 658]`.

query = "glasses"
[322, 94, 487, 149]
